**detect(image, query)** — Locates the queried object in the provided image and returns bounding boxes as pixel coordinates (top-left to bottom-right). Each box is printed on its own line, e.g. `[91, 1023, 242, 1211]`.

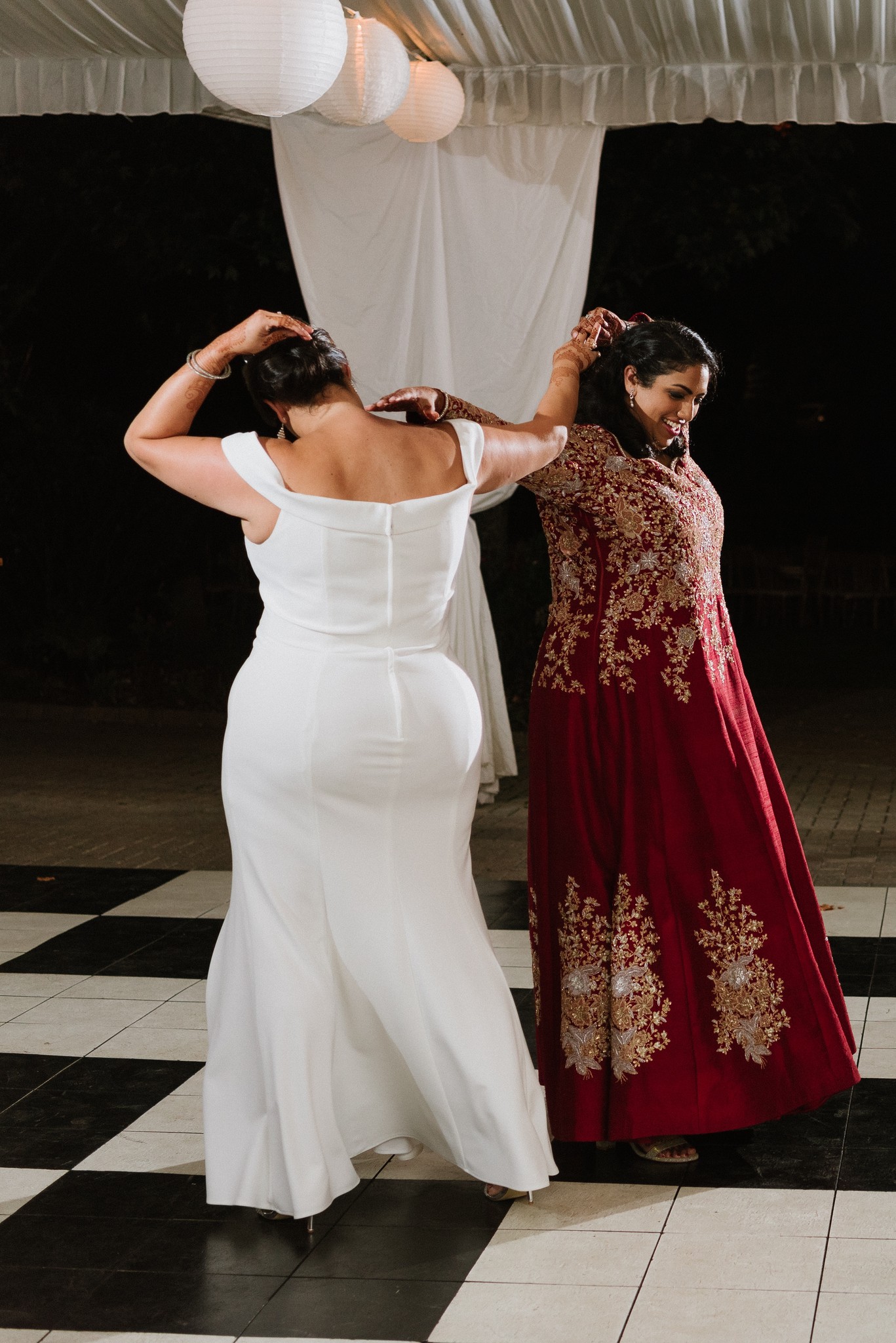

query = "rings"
[586, 338, 597, 349]
[585, 314, 593, 320]
[277, 312, 281, 314]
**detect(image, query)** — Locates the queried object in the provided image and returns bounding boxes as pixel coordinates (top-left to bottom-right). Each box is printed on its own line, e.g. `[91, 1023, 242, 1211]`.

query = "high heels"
[484, 1181, 533, 1205]
[256, 1208, 312, 1232]
[596, 1136, 698, 1163]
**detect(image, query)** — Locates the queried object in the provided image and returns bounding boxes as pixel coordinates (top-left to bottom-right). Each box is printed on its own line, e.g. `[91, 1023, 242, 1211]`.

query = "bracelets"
[187, 352, 227, 379]
[191, 349, 231, 379]
[435, 390, 448, 421]
[622, 320, 631, 330]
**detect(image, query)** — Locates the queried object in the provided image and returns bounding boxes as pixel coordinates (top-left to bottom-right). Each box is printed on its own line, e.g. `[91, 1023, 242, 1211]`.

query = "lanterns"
[182, 0, 348, 117]
[385, 61, 465, 143]
[311, 18, 408, 127]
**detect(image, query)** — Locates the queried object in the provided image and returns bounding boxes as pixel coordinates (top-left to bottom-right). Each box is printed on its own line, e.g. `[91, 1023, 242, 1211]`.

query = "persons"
[122, 308, 603, 1233]
[366, 308, 860, 1162]
